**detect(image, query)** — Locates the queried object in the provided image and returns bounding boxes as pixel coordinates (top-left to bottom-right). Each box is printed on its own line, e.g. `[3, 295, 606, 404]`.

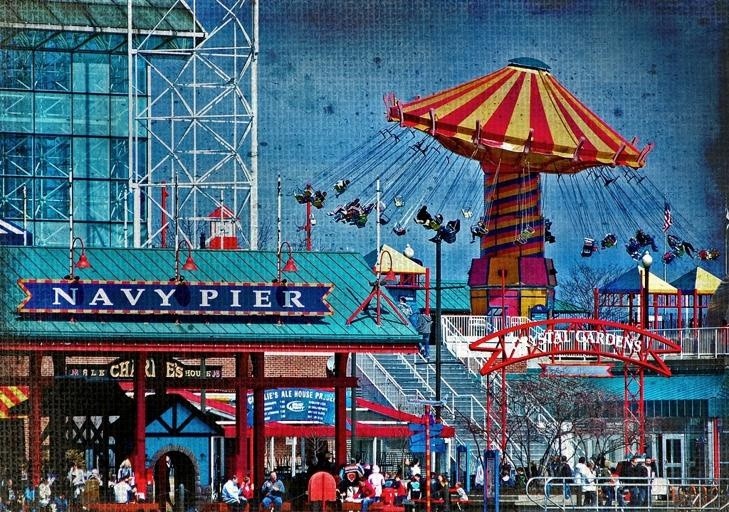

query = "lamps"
[378, 249, 397, 282]
[71, 237, 90, 279]
[278, 242, 297, 283]
[175, 239, 199, 282]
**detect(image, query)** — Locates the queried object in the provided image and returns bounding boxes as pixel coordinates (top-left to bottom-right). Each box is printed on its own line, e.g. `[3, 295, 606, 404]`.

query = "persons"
[415, 307, 434, 358]
[498, 457, 515, 489]
[429, 221, 458, 244]
[516, 467, 527, 493]
[333, 179, 350, 197]
[330, 203, 360, 222]
[625, 238, 640, 257]
[303, 182, 315, 204]
[539, 453, 654, 509]
[397, 296, 417, 321]
[636, 230, 659, 251]
[414, 205, 443, 230]
[668, 235, 697, 260]
[316, 191, 328, 202]
[470, 220, 488, 244]
[601, 233, 615, 249]
[328, 199, 360, 214]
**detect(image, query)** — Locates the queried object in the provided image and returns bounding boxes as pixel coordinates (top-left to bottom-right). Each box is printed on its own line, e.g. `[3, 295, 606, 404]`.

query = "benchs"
[341, 502, 385, 512]
[451, 495, 525, 512]
[91, 503, 159, 512]
[259, 502, 291, 512]
[218, 502, 249, 512]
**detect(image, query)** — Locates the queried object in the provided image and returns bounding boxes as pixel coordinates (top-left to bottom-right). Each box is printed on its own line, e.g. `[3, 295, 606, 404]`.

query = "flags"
[661, 199, 674, 232]
